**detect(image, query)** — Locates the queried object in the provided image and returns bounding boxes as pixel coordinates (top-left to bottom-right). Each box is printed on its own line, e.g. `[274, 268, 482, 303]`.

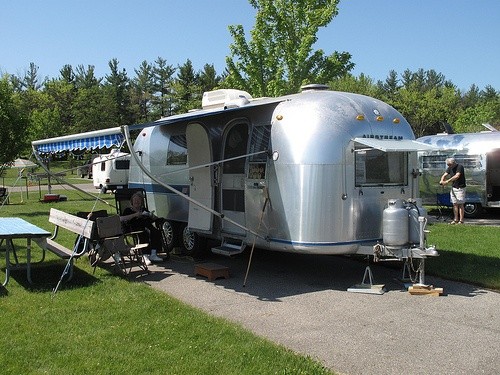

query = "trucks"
[31, 84, 440, 286]
[417, 121, 500, 214]
[93, 153, 131, 193]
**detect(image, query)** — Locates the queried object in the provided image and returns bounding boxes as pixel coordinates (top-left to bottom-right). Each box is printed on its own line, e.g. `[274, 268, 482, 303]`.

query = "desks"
[0, 216, 52, 289]
[22, 173, 67, 200]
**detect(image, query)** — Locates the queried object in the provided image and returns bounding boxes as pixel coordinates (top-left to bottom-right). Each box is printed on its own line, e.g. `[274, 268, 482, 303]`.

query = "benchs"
[32, 208, 95, 289]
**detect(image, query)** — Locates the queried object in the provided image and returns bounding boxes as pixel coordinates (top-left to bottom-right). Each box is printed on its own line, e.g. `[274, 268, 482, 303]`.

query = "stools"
[193, 261, 233, 281]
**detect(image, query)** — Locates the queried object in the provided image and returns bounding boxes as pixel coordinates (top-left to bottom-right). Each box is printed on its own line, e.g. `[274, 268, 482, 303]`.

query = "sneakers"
[149, 255, 163, 261]
[142, 256, 151, 265]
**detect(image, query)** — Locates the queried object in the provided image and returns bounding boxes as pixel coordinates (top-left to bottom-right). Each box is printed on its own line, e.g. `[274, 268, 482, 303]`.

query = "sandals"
[448, 220, 459, 224]
[455, 221, 464, 225]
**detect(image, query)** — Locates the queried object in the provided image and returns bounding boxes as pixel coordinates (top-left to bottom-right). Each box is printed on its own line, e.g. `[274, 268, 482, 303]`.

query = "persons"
[439, 157, 467, 225]
[121, 193, 163, 264]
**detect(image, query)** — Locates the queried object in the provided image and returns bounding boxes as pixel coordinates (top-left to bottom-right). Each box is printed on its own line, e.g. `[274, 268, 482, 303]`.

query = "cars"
[77, 160, 93, 179]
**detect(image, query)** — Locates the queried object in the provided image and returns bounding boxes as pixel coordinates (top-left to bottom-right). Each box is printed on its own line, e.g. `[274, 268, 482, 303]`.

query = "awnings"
[31, 97, 289, 154]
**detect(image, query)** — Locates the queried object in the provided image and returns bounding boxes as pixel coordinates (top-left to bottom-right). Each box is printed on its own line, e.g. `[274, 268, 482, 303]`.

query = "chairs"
[74, 187, 171, 282]
[0, 187, 10, 205]
[435, 192, 453, 221]
